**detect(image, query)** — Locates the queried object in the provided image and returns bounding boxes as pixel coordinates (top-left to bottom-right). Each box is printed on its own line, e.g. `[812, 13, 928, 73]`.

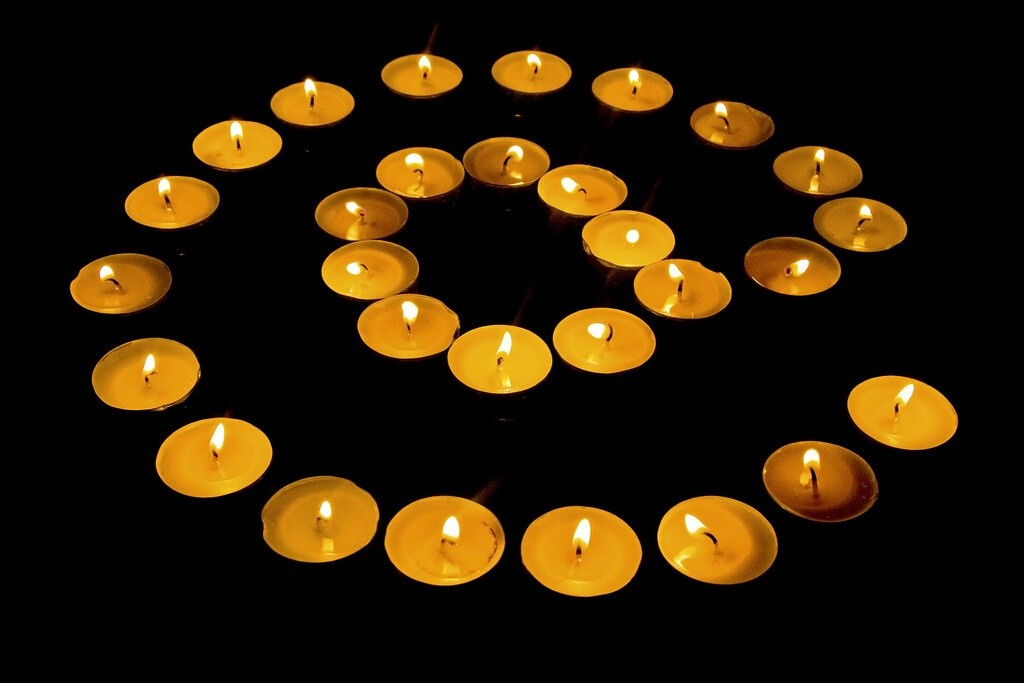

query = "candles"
[92, 338, 200, 409]
[193, 119, 282, 172]
[813, 197, 908, 253]
[746, 237, 842, 296]
[523, 507, 641, 598]
[69, 253, 173, 315]
[846, 373, 958, 450]
[691, 103, 775, 148]
[382, 55, 463, 98]
[763, 439, 879, 522]
[657, 495, 777, 586]
[270, 78, 355, 127]
[591, 67, 673, 112]
[775, 146, 862, 195]
[125, 175, 218, 230]
[262, 474, 378, 563]
[491, 52, 571, 92]
[155, 418, 272, 501]
[314, 137, 732, 396]
[384, 496, 504, 585]
